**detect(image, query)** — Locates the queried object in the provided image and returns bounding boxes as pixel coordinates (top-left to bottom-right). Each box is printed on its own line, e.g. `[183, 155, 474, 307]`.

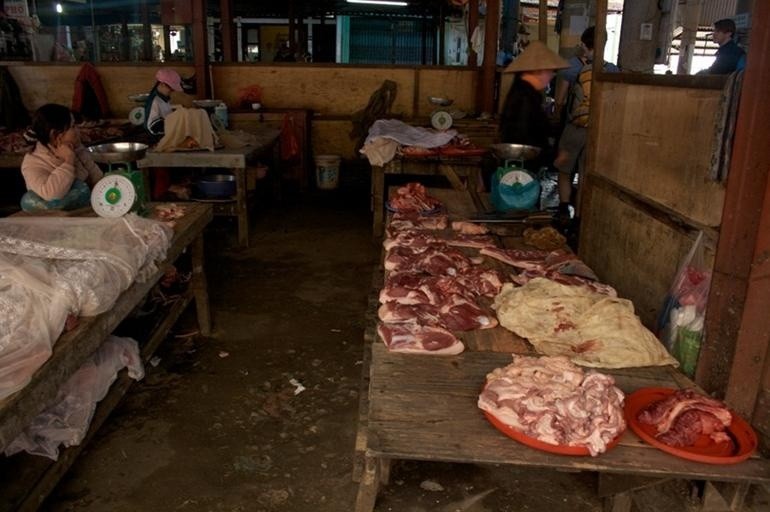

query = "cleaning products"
[215, 103, 229, 127]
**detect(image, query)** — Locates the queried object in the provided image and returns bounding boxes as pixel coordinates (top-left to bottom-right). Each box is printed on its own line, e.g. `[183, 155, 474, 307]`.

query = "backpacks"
[566, 52, 608, 129]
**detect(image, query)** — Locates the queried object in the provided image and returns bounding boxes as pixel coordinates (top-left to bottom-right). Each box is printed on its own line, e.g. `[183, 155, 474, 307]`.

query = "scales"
[427, 96, 454, 131]
[127, 93, 151, 126]
[87, 143, 151, 218]
[488, 143, 541, 212]
[192, 99, 223, 117]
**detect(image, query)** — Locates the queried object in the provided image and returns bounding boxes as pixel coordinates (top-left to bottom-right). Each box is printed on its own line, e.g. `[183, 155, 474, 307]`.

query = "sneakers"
[552, 201, 575, 221]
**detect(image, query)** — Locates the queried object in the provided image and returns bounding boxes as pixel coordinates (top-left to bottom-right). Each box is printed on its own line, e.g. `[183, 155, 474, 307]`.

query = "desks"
[3, 197, 218, 510]
[370, 143, 484, 243]
[137, 125, 288, 250]
[0, 128, 124, 169]
[352, 182, 769, 510]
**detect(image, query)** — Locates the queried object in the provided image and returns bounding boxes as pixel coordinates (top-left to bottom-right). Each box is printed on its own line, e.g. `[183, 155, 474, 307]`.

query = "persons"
[693, 17, 746, 74]
[21, 102, 105, 202]
[554, 24, 614, 234]
[141, 69, 185, 137]
[491, 68, 555, 172]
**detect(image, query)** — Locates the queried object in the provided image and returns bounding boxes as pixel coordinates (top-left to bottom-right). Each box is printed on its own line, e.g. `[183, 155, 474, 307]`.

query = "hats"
[502, 39, 580, 74]
[155, 68, 184, 92]
